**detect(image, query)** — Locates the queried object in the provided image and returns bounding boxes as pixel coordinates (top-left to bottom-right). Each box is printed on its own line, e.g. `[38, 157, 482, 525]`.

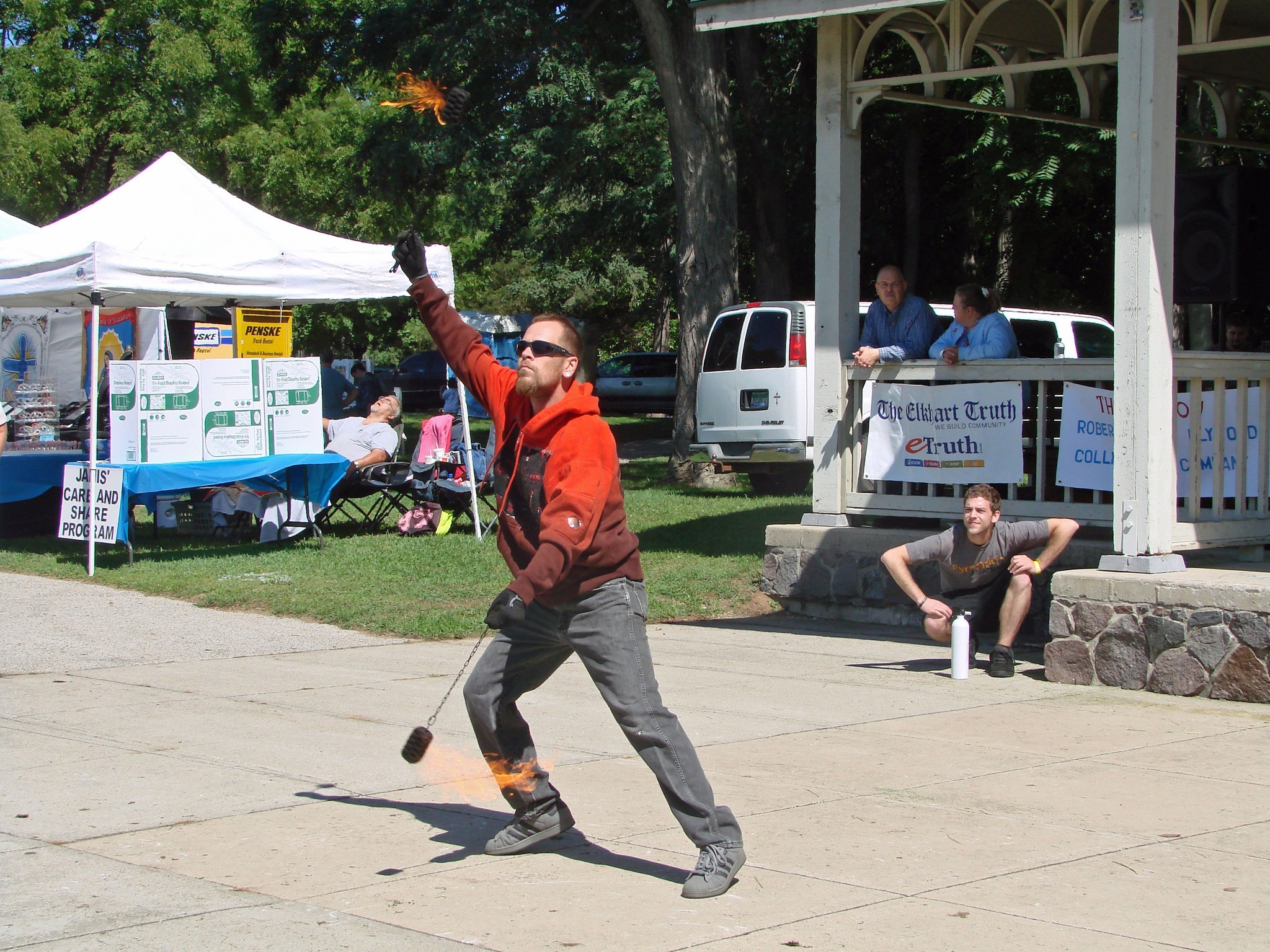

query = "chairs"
[213, 415, 498, 546]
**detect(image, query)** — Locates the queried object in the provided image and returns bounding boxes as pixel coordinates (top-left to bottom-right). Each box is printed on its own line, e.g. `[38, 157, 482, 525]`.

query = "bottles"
[446, 474, 455, 481]
[1054, 338, 1065, 359]
[951, 613, 969, 680]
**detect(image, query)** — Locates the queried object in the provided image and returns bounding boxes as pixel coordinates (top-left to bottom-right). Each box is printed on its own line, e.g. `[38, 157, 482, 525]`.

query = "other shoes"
[247, 528, 260, 543]
[910, 517, 940, 530]
[872, 515, 910, 528]
[947, 519, 963, 531]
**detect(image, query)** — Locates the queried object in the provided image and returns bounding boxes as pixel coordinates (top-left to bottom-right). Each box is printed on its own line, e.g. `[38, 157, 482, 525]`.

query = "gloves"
[484, 589, 526, 630]
[392, 230, 428, 282]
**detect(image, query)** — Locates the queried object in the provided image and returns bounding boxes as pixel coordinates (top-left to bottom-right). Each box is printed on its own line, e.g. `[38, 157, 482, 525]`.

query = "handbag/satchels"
[436, 509, 454, 536]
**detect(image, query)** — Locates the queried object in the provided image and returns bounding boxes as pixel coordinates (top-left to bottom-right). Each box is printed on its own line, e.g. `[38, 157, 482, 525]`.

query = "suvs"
[595, 352, 679, 413]
[390, 351, 452, 399]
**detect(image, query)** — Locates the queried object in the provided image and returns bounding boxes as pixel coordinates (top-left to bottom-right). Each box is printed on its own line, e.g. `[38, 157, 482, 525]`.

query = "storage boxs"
[171, 500, 253, 532]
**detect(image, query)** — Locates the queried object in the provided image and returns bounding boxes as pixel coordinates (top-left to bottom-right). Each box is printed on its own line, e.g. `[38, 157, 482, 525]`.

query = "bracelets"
[1033, 560, 1041, 575]
[917, 596, 928, 609]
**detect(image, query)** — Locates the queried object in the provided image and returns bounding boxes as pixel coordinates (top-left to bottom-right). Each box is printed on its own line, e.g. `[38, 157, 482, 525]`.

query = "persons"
[851, 264, 944, 529]
[1203, 317, 1259, 392]
[0, 401, 8, 457]
[322, 394, 402, 487]
[391, 228, 746, 899]
[439, 377, 461, 418]
[928, 283, 1030, 496]
[880, 484, 1079, 677]
[318, 349, 382, 447]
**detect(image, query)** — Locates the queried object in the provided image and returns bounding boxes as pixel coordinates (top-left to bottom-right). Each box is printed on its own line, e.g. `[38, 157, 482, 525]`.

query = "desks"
[0, 450, 109, 504]
[67, 453, 351, 569]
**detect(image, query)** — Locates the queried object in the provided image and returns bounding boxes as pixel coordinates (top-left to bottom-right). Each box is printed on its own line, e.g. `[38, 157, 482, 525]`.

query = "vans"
[687, 300, 1114, 498]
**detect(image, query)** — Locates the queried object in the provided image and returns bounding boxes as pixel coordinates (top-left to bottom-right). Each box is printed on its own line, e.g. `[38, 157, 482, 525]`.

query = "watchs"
[353, 460, 359, 470]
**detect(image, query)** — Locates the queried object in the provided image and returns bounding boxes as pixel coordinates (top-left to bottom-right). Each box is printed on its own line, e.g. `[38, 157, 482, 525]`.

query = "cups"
[425, 455, 436, 464]
[438, 455, 451, 463]
[432, 448, 445, 462]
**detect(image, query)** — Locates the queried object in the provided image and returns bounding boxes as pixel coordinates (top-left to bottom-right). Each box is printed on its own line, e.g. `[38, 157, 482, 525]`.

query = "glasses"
[876, 281, 904, 289]
[515, 340, 575, 356]
[379, 396, 395, 415]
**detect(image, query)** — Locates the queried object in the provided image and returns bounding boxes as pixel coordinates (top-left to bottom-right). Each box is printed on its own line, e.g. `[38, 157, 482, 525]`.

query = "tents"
[0, 152, 482, 576]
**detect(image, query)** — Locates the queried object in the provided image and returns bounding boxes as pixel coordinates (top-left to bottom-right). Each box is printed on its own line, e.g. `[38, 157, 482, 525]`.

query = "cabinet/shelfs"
[14, 379, 60, 441]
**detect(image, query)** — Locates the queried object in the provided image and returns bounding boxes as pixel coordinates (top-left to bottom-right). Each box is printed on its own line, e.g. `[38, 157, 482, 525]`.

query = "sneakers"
[988, 642, 1015, 677]
[681, 844, 746, 899]
[969, 633, 979, 669]
[483, 798, 576, 856]
